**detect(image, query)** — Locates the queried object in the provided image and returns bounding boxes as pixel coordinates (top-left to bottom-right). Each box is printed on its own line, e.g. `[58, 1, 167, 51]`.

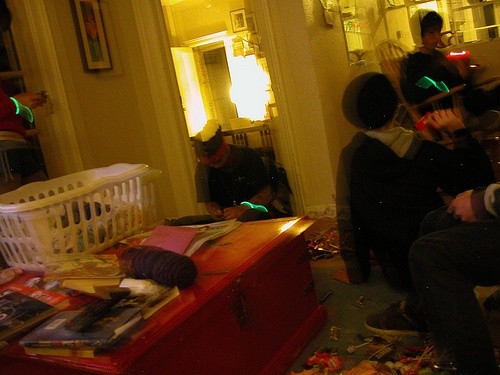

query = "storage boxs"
[0, 163, 149, 273]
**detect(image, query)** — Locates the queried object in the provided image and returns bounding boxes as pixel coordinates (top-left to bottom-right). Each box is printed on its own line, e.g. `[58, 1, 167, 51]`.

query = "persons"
[190, 118, 293, 222]
[334, 73, 481, 291]
[0, 88, 50, 138]
[398, 9, 493, 148]
[362, 176, 500, 375]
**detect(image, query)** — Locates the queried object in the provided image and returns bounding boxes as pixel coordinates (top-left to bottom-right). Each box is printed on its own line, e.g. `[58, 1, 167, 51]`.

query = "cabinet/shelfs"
[379, 0, 500, 59]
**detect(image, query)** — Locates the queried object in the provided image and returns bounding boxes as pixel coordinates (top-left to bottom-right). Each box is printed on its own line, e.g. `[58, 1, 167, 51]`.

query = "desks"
[0, 214, 329, 375]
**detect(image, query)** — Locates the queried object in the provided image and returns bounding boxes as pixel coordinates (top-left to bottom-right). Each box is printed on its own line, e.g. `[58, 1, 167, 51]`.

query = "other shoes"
[364, 299, 433, 337]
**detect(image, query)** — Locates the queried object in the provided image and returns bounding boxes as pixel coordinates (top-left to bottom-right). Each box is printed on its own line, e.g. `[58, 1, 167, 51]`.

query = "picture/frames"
[68, 0, 114, 74]
[230, 8, 259, 35]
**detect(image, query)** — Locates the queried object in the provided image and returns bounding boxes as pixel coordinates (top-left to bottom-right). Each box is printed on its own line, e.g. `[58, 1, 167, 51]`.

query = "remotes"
[63, 287, 131, 332]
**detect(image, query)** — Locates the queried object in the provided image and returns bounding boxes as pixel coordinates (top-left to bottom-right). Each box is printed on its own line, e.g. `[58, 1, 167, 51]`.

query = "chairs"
[376, 42, 500, 150]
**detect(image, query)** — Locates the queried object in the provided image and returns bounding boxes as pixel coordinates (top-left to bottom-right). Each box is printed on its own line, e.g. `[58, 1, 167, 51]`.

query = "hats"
[191, 120, 224, 157]
